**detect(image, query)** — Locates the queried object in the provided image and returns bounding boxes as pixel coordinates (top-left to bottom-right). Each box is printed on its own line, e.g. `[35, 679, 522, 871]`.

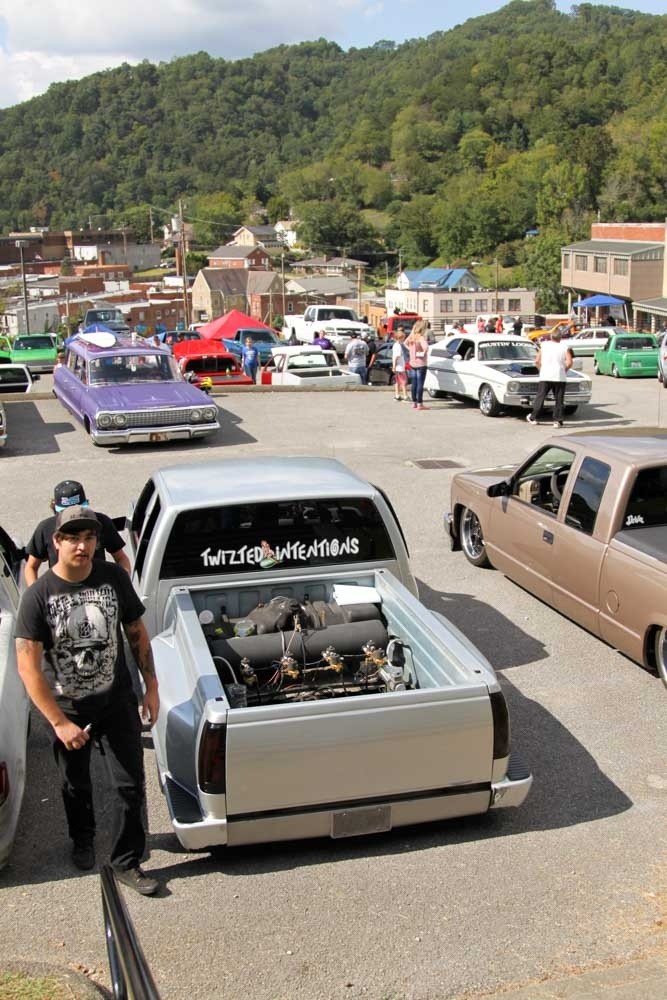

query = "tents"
[571, 294, 630, 333]
[195, 310, 278, 342]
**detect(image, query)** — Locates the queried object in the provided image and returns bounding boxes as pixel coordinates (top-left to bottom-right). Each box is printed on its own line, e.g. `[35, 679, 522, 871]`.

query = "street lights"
[470, 257, 499, 312]
[16, 239, 29, 334]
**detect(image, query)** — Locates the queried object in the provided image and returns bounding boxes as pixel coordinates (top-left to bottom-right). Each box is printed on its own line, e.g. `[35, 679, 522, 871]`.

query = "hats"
[54, 480, 88, 513]
[56, 505, 102, 533]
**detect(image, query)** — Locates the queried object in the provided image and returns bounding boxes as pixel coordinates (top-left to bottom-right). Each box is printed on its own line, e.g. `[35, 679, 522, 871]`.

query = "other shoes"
[553, 422, 562, 428]
[112, 867, 160, 894]
[527, 414, 538, 425]
[417, 404, 430, 410]
[71, 833, 97, 871]
[394, 396, 401, 401]
[404, 397, 412, 402]
[412, 403, 417, 408]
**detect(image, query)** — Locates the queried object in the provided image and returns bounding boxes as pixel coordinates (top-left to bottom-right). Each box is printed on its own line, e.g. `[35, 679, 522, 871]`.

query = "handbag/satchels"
[415, 335, 425, 357]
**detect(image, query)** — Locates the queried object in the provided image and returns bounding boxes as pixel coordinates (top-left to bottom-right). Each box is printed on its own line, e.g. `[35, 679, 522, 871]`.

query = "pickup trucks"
[107, 454, 533, 851]
[422, 332, 593, 418]
[172, 339, 254, 388]
[280, 304, 372, 354]
[376, 315, 422, 342]
[443, 426, 667, 689]
[261, 345, 362, 387]
[443, 314, 536, 337]
[221, 328, 286, 368]
[0, 362, 41, 395]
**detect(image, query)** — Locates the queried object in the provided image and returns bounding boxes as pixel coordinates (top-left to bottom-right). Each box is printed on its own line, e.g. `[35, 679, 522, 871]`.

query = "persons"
[527, 327, 573, 428]
[344, 332, 377, 386]
[241, 337, 261, 385]
[513, 316, 523, 335]
[313, 331, 335, 361]
[13, 480, 161, 894]
[477, 314, 504, 334]
[391, 319, 431, 410]
[150, 333, 185, 355]
[447, 321, 467, 337]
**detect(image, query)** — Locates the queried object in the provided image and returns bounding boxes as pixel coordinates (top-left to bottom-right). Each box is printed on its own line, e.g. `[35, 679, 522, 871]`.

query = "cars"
[77, 307, 132, 338]
[52, 338, 222, 447]
[368, 342, 416, 386]
[594, 333, 659, 378]
[0, 397, 8, 447]
[0, 333, 66, 372]
[0, 526, 33, 875]
[145, 323, 201, 355]
[657, 332, 667, 388]
[528, 320, 626, 357]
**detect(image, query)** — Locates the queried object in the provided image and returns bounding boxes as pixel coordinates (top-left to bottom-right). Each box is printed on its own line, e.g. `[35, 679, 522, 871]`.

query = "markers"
[84, 723, 92, 732]
